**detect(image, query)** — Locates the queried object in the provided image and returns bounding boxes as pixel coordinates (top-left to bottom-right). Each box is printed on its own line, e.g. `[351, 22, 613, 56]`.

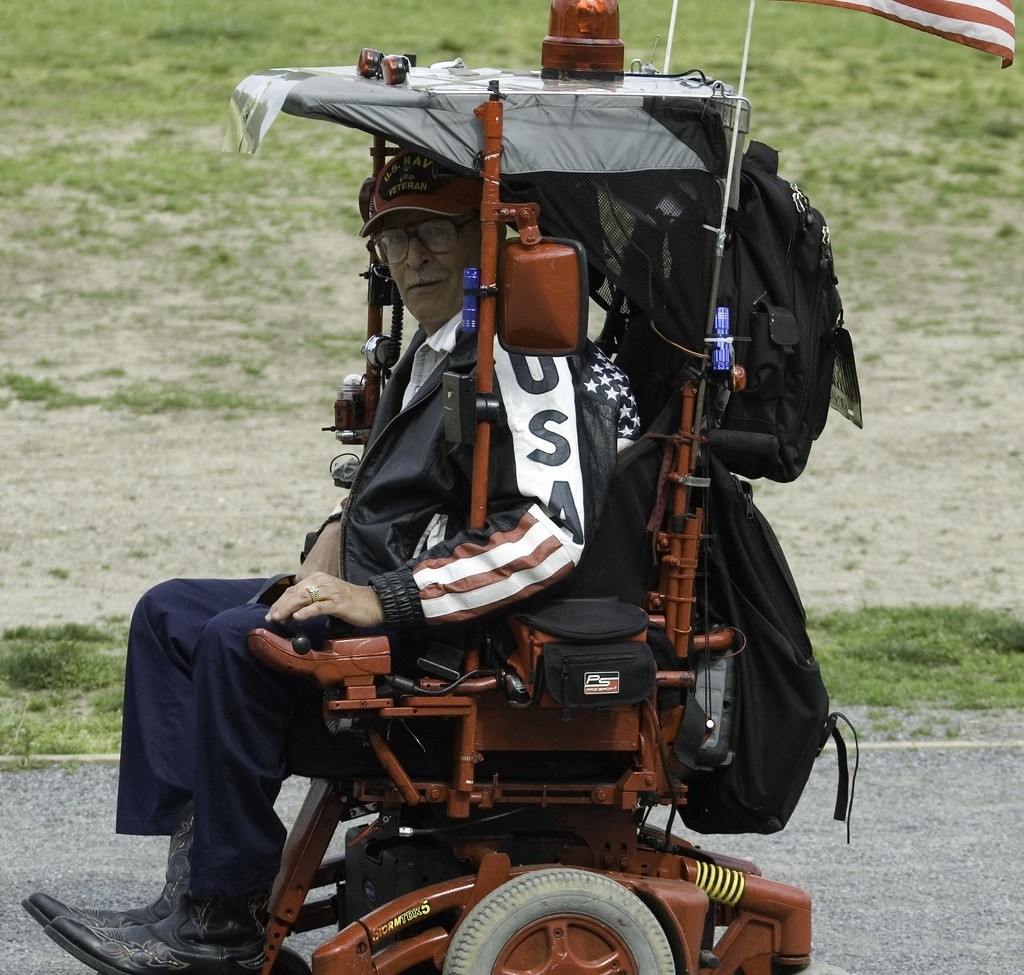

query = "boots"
[20, 797, 195, 929]
[44, 873, 268, 975]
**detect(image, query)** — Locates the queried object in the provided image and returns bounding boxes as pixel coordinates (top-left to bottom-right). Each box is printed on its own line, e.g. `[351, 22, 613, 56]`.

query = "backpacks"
[596, 97, 863, 483]
[563, 376, 860, 845]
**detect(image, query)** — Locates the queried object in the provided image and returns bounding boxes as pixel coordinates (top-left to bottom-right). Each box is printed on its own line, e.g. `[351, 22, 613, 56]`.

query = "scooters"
[234, 0, 811, 975]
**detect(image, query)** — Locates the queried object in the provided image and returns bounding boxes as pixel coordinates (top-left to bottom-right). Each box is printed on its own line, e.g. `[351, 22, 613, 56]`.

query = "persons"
[20, 146, 659, 975]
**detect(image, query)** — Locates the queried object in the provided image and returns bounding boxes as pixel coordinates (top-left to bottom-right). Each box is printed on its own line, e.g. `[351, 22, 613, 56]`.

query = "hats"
[358, 149, 484, 238]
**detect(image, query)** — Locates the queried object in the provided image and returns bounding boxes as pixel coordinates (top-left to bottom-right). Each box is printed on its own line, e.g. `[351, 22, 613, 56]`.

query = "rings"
[307, 585, 319, 603]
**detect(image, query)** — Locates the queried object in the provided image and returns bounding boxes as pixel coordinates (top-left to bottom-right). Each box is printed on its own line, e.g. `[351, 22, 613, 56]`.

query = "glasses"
[368, 213, 485, 266]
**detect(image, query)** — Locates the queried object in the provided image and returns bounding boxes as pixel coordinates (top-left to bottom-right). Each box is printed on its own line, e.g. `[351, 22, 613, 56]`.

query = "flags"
[791, 0, 1018, 69]
